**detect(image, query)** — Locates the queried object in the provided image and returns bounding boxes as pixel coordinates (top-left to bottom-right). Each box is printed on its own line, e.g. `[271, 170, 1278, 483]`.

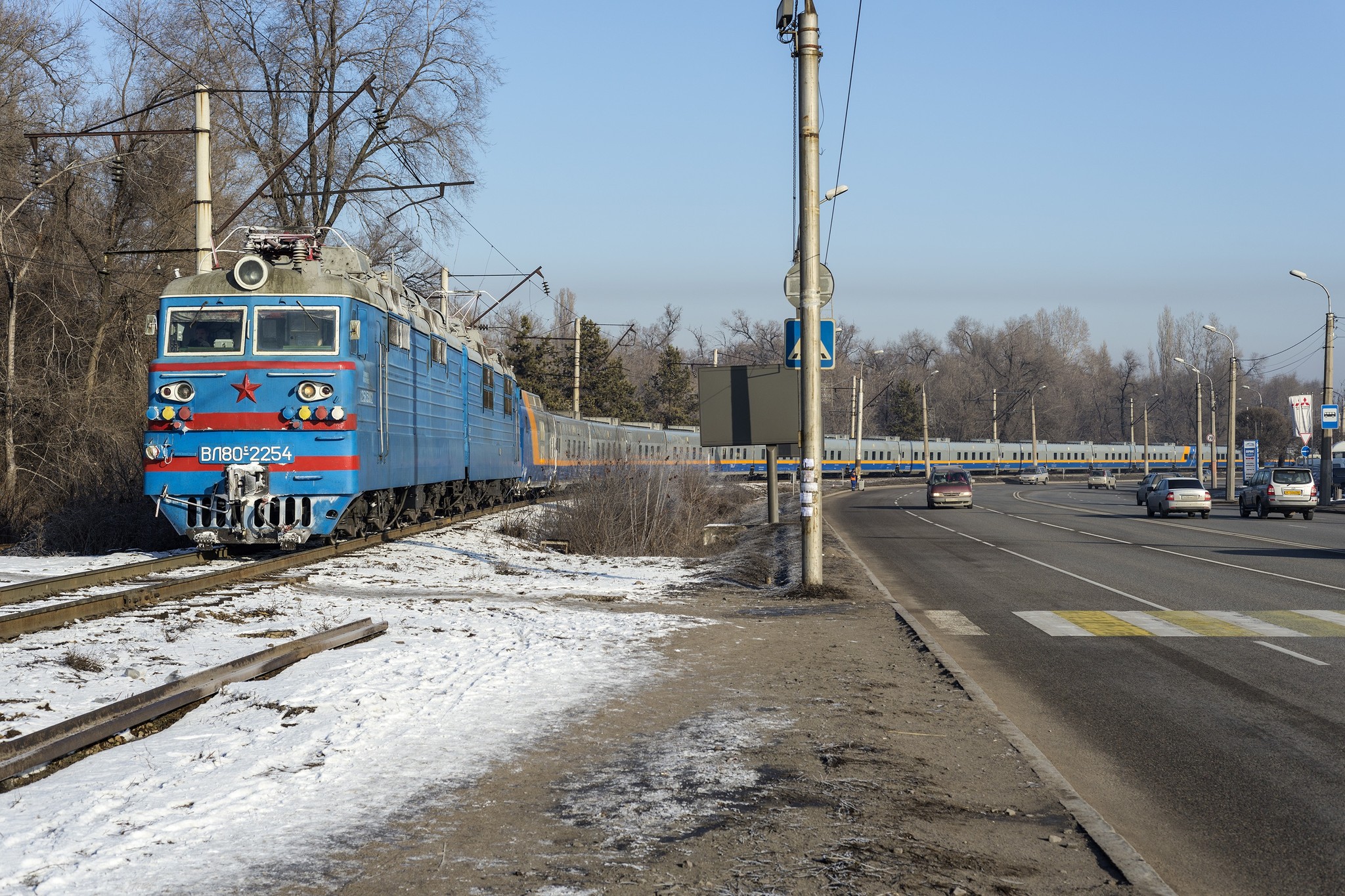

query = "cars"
[1145, 477, 1212, 519]
[1190, 469, 1211, 482]
[1087, 469, 1117, 490]
[1019, 466, 1049, 485]
[925, 464, 976, 509]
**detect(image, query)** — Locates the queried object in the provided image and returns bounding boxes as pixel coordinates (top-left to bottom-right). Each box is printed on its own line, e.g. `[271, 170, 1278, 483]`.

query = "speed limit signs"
[1206, 433, 1215, 442]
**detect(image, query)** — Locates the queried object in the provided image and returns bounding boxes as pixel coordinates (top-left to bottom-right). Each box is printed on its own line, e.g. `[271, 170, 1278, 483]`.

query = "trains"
[142, 220, 1246, 562]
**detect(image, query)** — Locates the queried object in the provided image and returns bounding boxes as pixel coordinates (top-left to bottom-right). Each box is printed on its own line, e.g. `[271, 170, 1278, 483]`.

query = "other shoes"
[852, 490, 855, 491]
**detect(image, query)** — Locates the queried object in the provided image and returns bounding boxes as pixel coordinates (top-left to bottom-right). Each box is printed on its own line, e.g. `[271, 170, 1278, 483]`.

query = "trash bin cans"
[858, 479, 865, 491]
[1333, 484, 1342, 499]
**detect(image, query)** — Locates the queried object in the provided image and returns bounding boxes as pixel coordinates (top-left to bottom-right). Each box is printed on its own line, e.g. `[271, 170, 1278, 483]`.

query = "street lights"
[1191, 367, 1217, 489]
[1203, 324, 1237, 501]
[1289, 268, 1335, 506]
[1143, 393, 1158, 477]
[921, 370, 939, 480]
[1030, 385, 1047, 466]
[1241, 385, 1263, 438]
[854, 349, 885, 483]
[1174, 356, 1204, 487]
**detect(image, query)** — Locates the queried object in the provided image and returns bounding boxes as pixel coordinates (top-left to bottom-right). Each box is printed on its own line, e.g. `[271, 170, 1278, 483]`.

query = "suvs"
[1136, 473, 1185, 506]
[1238, 466, 1318, 520]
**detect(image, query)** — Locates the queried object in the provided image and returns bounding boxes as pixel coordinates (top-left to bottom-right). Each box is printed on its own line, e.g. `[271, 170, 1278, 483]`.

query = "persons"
[187, 325, 218, 348]
[850, 468, 857, 491]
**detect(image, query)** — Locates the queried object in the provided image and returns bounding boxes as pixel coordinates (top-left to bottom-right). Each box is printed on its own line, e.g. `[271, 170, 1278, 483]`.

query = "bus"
[1295, 454, 1345, 500]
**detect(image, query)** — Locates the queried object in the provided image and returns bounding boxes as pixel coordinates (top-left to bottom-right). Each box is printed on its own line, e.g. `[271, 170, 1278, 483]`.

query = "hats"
[852, 468, 855, 470]
